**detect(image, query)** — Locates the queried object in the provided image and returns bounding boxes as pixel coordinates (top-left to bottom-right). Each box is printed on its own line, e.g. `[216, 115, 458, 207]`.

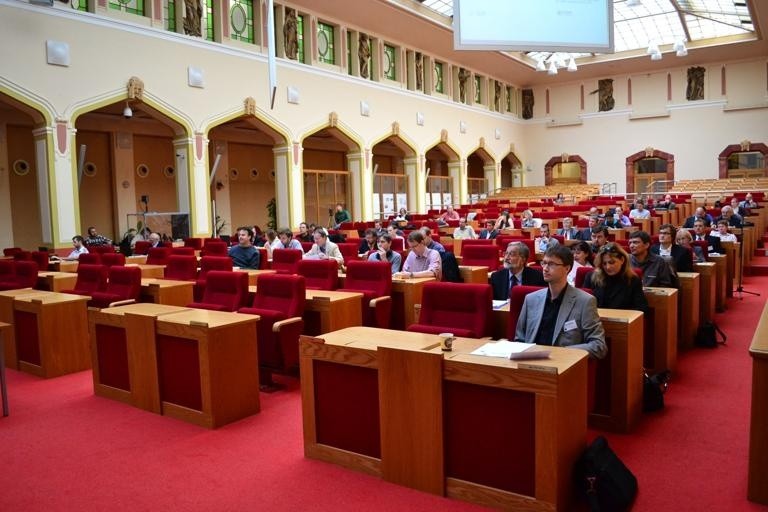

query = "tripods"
[730, 217, 760, 300]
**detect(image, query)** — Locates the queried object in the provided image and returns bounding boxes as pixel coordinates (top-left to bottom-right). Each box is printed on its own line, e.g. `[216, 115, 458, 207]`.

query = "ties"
[698, 236, 700, 241]
[509, 274, 519, 294]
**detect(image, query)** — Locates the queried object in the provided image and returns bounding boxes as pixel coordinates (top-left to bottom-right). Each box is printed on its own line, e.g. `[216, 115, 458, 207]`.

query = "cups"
[439, 333, 454, 351]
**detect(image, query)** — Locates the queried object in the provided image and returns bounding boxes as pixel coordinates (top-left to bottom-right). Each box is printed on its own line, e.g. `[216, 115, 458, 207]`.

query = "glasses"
[658, 231, 672, 236]
[407, 243, 421, 250]
[375, 240, 387, 244]
[539, 259, 566, 269]
[502, 251, 523, 258]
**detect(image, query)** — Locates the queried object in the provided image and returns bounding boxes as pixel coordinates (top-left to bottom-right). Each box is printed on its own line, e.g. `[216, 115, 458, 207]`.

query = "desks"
[758, 201, 768, 232]
[501, 226, 521, 235]
[623, 224, 638, 239]
[743, 215, 759, 255]
[504, 226, 541, 240]
[647, 214, 663, 237]
[640, 283, 679, 382]
[468, 207, 482, 216]
[733, 226, 750, 270]
[496, 202, 510, 209]
[563, 238, 587, 248]
[138, 275, 197, 305]
[490, 298, 510, 338]
[721, 238, 740, 295]
[0, 286, 91, 379]
[614, 200, 626, 212]
[666, 206, 679, 226]
[393, 274, 435, 329]
[596, 204, 609, 214]
[629, 216, 648, 235]
[587, 306, 645, 433]
[463, 220, 479, 231]
[126, 252, 147, 265]
[441, 236, 460, 256]
[245, 281, 364, 335]
[574, 211, 587, 222]
[746, 294, 768, 505]
[297, 326, 590, 512]
[608, 226, 625, 241]
[539, 217, 558, 234]
[750, 207, 766, 245]
[655, 210, 670, 227]
[46, 256, 79, 273]
[693, 260, 717, 325]
[678, 204, 691, 222]
[709, 252, 728, 312]
[89, 302, 260, 431]
[675, 270, 705, 344]
[743, 220, 755, 259]
[437, 224, 457, 236]
[674, 204, 685, 225]
[235, 265, 277, 283]
[685, 198, 697, 215]
[336, 229, 359, 241]
[456, 262, 489, 284]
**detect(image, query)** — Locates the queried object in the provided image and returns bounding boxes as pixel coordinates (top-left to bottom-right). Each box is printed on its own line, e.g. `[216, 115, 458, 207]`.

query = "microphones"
[744, 200, 749, 207]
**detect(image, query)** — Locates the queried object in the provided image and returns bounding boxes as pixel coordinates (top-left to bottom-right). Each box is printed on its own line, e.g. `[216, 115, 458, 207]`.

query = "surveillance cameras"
[123, 102, 133, 119]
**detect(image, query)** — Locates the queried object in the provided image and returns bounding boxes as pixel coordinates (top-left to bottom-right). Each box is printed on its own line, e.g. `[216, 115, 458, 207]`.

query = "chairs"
[90, 265, 143, 307]
[488, 184, 600, 201]
[258, 247, 268, 270]
[575, 266, 594, 287]
[206, 241, 228, 257]
[382, 222, 391, 228]
[428, 210, 440, 215]
[487, 207, 499, 212]
[424, 221, 438, 232]
[751, 190, 765, 197]
[354, 221, 368, 234]
[348, 235, 365, 250]
[460, 244, 500, 270]
[601, 200, 616, 206]
[402, 229, 413, 234]
[203, 239, 221, 250]
[76, 252, 99, 272]
[472, 204, 485, 209]
[726, 195, 745, 202]
[513, 219, 524, 228]
[334, 242, 359, 264]
[612, 196, 626, 201]
[440, 209, 449, 215]
[294, 257, 339, 291]
[658, 198, 673, 203]
[550, 234, 565, 246]
[735, 191, 748, 198]
[236, 272, 305, 392]
[96, 245, 113, 253]
[30, 249, 48, 272]
[387, 238, 404, 256]
[485, 212, 498, 220]
[2, 248, 22, 257]
[500, 238, 535, 262]
[576, 204, 596, 212]
[663, 194, 676, 197]
[668, 177, 768, 194]
[458, 213, 466, 219]
[719, 200, 729, 207]
[489, 199, 498, 203]
[459, 238, 491, 258]
[678, 194, 691, 199]
[136, 241, 152, 256]
[496, 232, 523, 248]
[555, 210, 572, 223]
[577, 200, 597, 206]
[487, 203, 497, 207]
[184, 237, 202, 249]
[617, 237, 629, 252]
[160, 253, 197, 285]
[146, 247, 170, 265]
[298, 240, 312, 253]
[0, 257, 16, 290]
[692, 239, 709, 261]
[14, 249, 31, 260]
[12, 260, 39, 289]
[455, 208, 467, 213]
[705, 209, 721, 222]
[554, 204, 575, 213]
[576, 218, 589, 229]
[86, 246, 96, 253]
[170, 246, 194, 256]
[387, 216, 401, 220]
[409, 214, 422, 222]
[461, 205, 471, 210]
[539, 211, 555, 219]
[474, 212, 484, 223]
[500, 200, 510, 205]
[447, 219, 460, 226]
[102, 253, 125, 267]
[423, 214, 434, 221]
[196, 256, 233, 287]
[407, 221, 422, 228]
[339, 221, 354, 231]
[271, 247, 302, 275]
[338, 258, 393, 327]
[506, 284, 593, 340]
[58, 262, 108, 297]
[671, 198, 685, 204]
[185, 269, 250, 312]
[410, 278, 494, 339]
[368, 221, 375, 229]
[593, 194, 613, 200]
[430, 234, 440, 241]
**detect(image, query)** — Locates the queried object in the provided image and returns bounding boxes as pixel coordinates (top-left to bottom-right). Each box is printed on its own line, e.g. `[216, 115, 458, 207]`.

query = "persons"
[651, 224, 687, 273]
[392, 232, 442, 281]
[478, 220, 498, 239]
[601, 211, 622, 229]
[416, 227, 446, 257]
[227, 226, 260, 268]
[279, 228, 303, 256]
[613, 207, 632, 227]
[535, 224, 559, 252]
[588, 207, 606, 219]
[710, 222, 737, 243]
[522, 210, 536, 227]
[684, 208, 714, 229]
[727, 198, 750, 217]
[389, 221, 404, 239]
[250, 226, 265, 246]
[119, 228, 136, 254]
[659, 195, 675, 210]
[559, 218, 581, 241]
[593, 243, 647, 315]
[359, 229, 379, 258]
[739, 194, 756, 208]
[394, 208, 410, 224]
[148, 234, 163, 249]
[629, 200, 650, 221]
[645, 198, 656, 218]
[334, 203, 350, 226]
[717, 207, 742, 226]
[131, 228, 152, 248]
[570, 242, 593, 270]
[675, 229, 703, 263]
[294, 222, 310, 242]
[386, 225, 405, 251]
[492, 243, 544, 302]
[514, 246, 608, 366]
[584, 217, 605, 239]
[626, 232, 672, 288]
[454, 218, 476, 240]
[714, 196, 727, 208]
[373, 221, 387, 237]
[262, 230, 284, 261]
[554, 193, 563, 205]
[304, 230, 344, 264]
[690, 220, 720, 254]
[441, 205, 460, 221]
[302, 228, 308, 240]
[85, 226, 112, 245]
[308, 223, 316, 232]
[495, 211, 514, 230]
[366, 232, 401, 274]
[52, 235, 88, 260]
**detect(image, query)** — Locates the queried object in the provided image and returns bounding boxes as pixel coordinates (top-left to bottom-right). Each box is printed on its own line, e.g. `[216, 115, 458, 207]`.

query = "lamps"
[555, 54, 565, 69]
[536, 52, 546, 71]
[647, 39, 662, 59]
[567, 57, 577, 74]
[548, 57, 555, 75]
[672, 36, 689, 56]
